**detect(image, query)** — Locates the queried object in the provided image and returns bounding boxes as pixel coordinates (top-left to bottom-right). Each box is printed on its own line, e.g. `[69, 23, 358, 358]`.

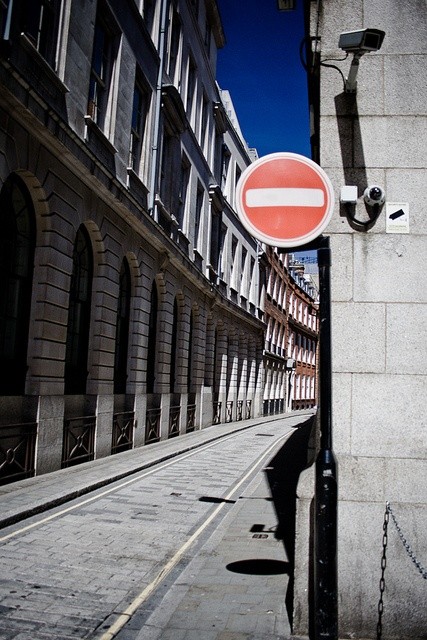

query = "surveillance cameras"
[363, 184, 384, 205]
[338, 28, 385, 90]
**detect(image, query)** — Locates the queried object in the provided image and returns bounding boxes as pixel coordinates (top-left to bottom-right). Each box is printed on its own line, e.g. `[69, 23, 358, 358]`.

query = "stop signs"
[234, 152, 334, 248]
